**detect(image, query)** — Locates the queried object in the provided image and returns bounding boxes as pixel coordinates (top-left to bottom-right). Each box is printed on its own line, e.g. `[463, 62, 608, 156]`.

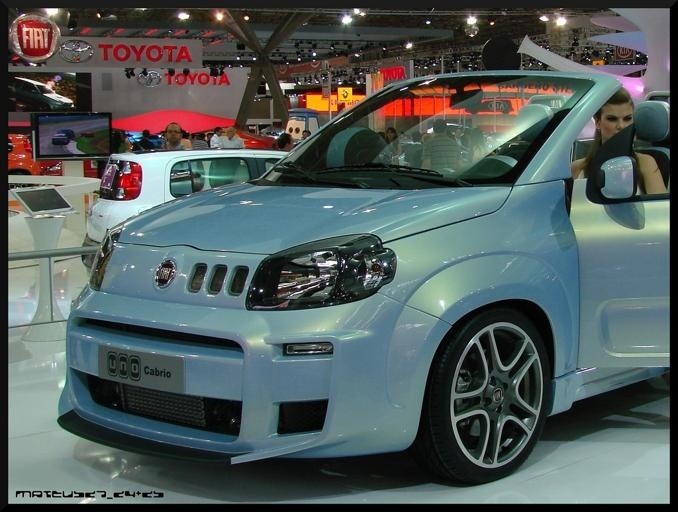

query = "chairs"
[628, 98, 670, 192]
[514, 102, 552, 159]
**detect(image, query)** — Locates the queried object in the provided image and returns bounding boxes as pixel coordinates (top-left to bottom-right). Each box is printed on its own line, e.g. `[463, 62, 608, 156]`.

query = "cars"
[259, 125, 284, 139]
[53, 128, 75, 145]
[51, 66, 671, 483]
[8, 133, 63, 192]
[397, 142, 468, 167]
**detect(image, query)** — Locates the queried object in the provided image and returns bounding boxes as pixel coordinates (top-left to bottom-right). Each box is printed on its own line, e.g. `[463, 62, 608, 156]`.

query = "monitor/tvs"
[31, 112, 112, 161]
[11, 186, 72, 215]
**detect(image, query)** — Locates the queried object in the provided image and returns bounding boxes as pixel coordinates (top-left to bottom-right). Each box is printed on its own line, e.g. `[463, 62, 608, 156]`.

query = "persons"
[377, 118, 498, 174]
[112, 121, 245, 153]
[277, 132, 294, 152]
[570, 86, 667, 195]
[302, 129, 311, 139]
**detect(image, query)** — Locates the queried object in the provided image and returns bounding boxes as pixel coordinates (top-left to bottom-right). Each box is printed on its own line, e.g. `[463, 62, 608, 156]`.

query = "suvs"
[15, 77, 74, 110]
[83, 148, 289, 280]
